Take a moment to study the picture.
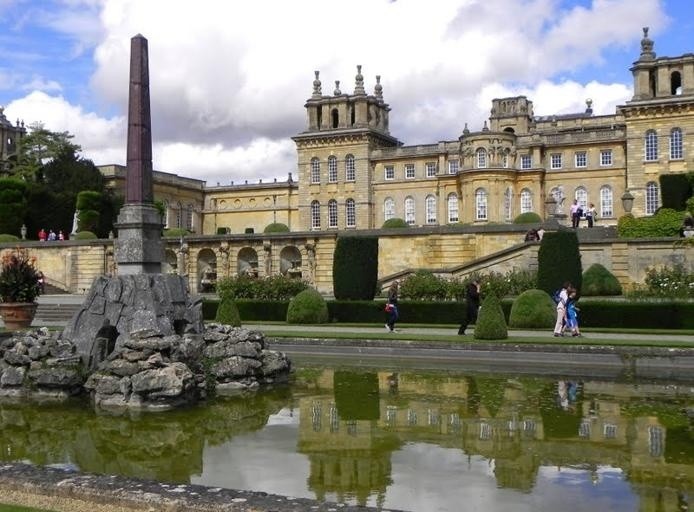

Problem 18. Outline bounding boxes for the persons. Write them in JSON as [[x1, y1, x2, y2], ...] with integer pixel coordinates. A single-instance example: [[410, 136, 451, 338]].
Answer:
[[553, 280, 580, 337], [559, 287, 581, 337], [464, 375, 481, 420], [569, 199, 582, 227], [384, 280, 401, 334], [46, 229, 56, 241], [534, 228, 546, 242], [457, 277, 481, 335], [385, 371, 400, 398], [556, 379, 570, 412], [567, 380, 579, 415], [37, 229, 46, 241], [585, 204, 598, 227], [56, 230, 64, 241], [35, 270, 46, 296], [524, 228, 540, 243]]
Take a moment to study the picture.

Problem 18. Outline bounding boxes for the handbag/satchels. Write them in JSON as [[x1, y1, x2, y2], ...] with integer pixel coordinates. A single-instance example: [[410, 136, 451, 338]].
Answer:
[[385, 303, 394, 313]]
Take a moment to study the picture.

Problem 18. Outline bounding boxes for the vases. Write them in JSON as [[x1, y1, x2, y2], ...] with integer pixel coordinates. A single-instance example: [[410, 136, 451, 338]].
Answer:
[[0, 304, 39, 330]]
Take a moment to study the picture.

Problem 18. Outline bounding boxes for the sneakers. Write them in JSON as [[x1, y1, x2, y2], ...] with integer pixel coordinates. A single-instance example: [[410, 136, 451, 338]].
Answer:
[[554, 332, 581, 337], [385, 323, 398, 333]]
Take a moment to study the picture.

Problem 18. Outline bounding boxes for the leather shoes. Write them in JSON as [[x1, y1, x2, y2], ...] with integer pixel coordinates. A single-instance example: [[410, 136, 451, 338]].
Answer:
[[458, 332, 465, 335]]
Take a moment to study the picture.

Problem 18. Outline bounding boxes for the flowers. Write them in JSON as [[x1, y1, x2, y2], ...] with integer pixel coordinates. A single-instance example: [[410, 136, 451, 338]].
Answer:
[[0, 245, 44, 302]]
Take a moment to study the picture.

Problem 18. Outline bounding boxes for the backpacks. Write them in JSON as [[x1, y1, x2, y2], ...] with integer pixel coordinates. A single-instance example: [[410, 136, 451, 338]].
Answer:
[[552, 288, 562, 304]]
